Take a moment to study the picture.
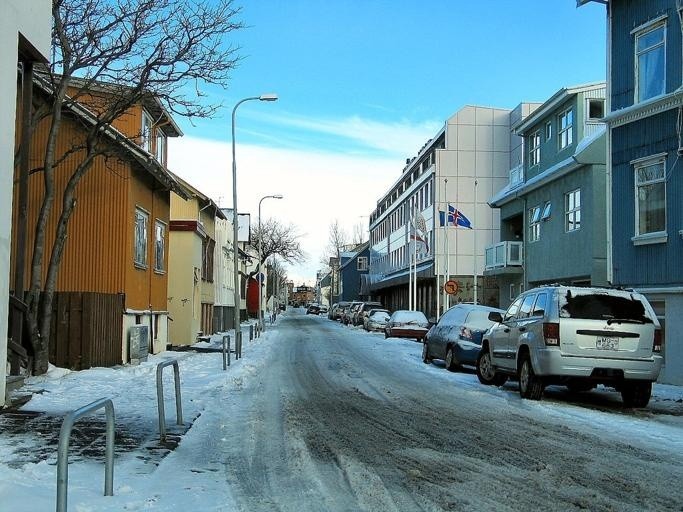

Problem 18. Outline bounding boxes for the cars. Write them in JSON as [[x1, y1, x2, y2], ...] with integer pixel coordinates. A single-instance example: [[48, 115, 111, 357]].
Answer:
[[292, 301, 299, 308], [279, 302, 286, 310], [307, 303, 327, 315]]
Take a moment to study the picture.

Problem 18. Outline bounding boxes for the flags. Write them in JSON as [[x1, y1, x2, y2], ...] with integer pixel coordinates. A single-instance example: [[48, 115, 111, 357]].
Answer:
[[408, 222, 425, 242], [439, 212, 453, 227], [414, 208, 429, 253], [446, 204, 472, 229]]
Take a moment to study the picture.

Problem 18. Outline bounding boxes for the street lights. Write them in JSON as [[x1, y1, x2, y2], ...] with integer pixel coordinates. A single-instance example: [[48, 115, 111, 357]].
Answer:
[[231, 96, 277, 352], [259, 195, 282, 324]]
